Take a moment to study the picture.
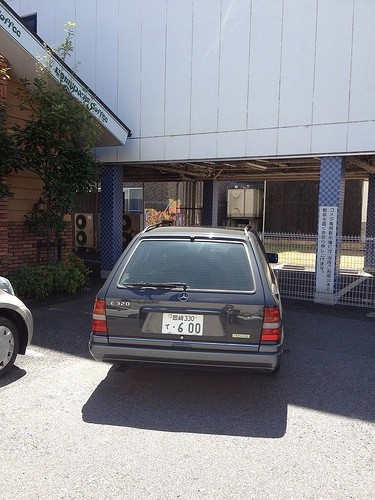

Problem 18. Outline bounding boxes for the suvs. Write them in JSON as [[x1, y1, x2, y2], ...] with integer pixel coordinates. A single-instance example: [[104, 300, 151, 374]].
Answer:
[[88, 222, 284, 375]]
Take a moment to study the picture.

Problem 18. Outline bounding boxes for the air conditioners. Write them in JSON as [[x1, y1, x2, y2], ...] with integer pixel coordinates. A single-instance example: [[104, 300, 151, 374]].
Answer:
[[73, 211, 96, 251]]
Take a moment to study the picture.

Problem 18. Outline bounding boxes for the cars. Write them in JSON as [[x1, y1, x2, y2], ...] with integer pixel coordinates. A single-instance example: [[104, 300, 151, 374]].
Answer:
[[0, 276, 34, 378]]
